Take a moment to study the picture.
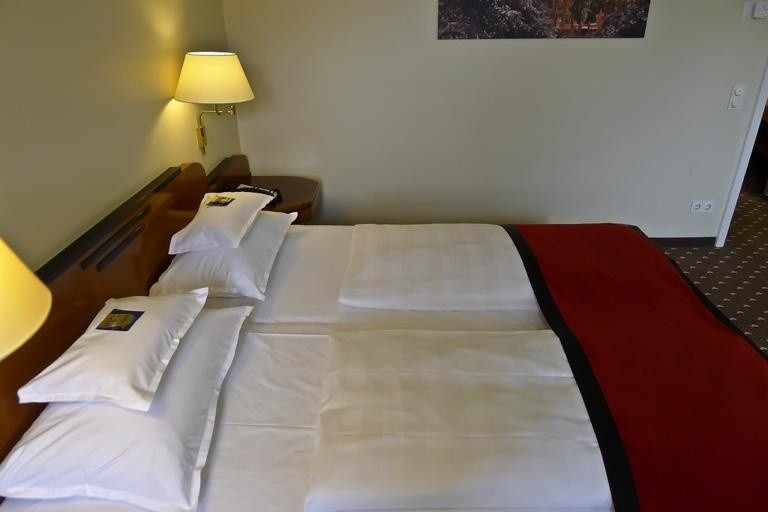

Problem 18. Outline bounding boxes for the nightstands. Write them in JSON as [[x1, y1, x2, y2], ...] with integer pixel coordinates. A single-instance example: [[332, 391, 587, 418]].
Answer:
[[207, 155, 322, 225]]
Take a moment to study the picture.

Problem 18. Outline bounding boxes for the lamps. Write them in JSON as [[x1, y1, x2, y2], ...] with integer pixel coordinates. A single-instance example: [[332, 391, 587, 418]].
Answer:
[[172, 52, 257, 156], [0, 239, 52, 361]]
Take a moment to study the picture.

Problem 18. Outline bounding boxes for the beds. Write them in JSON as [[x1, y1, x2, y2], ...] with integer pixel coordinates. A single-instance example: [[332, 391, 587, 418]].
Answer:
[[0, 162, 768, 511]]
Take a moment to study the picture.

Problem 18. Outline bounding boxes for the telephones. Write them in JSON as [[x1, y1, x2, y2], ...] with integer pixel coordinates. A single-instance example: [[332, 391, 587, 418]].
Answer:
[[223, 182, 283, 207]]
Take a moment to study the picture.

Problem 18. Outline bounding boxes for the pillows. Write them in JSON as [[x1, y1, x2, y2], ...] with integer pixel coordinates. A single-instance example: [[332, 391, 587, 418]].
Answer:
[[170, 192, 275, 254], [0, 306, 256, 512], [149, 213, 294, 299], [15, 283, 209, 415]]
